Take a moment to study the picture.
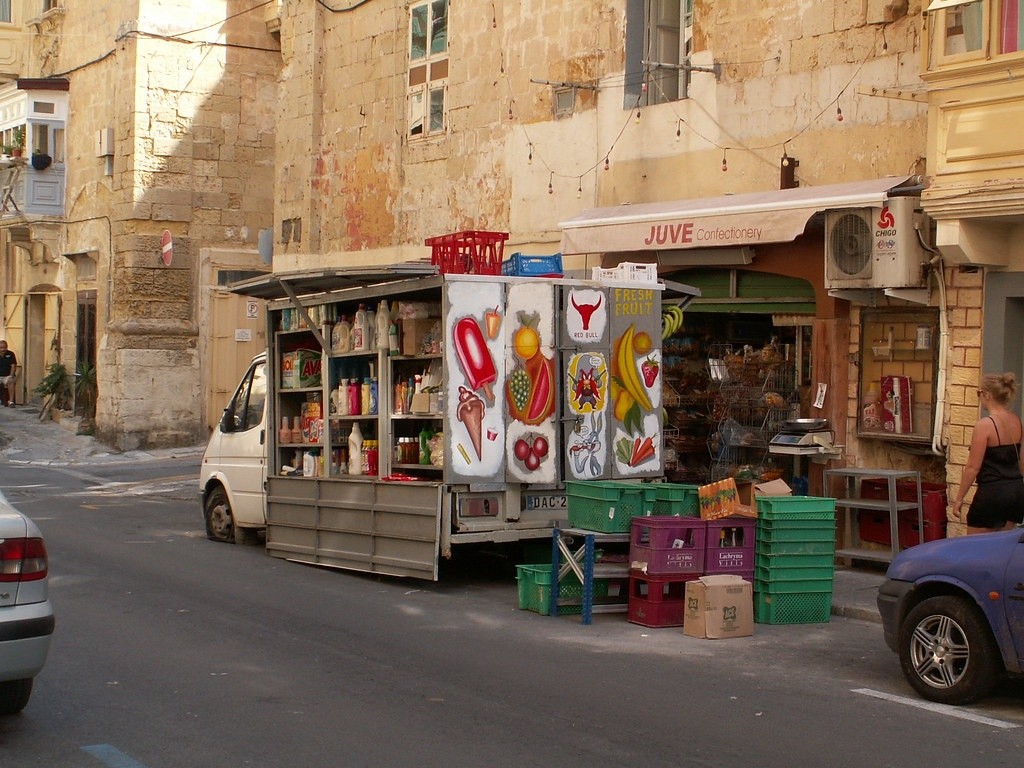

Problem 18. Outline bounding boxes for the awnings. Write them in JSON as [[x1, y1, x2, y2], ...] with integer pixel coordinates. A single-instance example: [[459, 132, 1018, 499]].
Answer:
[[559, 176, 917, 255], [215, 264, 439, 355]]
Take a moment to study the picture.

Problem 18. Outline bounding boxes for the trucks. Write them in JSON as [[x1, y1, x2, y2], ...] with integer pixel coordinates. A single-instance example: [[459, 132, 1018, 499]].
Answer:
[[198, 265, 701, 582]]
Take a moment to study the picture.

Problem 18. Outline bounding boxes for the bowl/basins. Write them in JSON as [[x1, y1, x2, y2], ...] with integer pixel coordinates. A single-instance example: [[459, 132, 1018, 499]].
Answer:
[[780, 418, 827, 430]]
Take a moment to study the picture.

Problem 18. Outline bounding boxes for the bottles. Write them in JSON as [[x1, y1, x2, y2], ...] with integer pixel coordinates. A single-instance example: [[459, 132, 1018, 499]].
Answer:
[[863, 383, 881, 430], [398, 437, 419, 464], [888, 326, 895, 349], [280, 416, 302, 443], [419, 428, 435, 464], [389, 319, 399, 356], [361, 440, 378, 476], [332, 450, 348, 473]]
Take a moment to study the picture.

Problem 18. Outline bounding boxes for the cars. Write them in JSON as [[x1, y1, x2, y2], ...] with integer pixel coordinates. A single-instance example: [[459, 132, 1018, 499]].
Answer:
[[876, 527, 1024, 704], [0, 487, 55, 713]]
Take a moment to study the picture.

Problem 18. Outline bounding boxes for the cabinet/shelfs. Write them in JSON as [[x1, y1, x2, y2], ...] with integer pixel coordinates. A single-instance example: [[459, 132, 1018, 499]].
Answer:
[[663, 335, 813, 483], [274, 312, 443, 479], [823, 467, 924, 563], [551, 528, 650, 625]]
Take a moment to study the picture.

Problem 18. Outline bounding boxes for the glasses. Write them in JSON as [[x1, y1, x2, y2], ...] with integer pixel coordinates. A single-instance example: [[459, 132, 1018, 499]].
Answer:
[[977, 390, 993, 401]]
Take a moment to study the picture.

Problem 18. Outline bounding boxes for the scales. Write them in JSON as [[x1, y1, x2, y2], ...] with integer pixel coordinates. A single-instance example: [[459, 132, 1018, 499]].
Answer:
[[769, 418, 834, 446]]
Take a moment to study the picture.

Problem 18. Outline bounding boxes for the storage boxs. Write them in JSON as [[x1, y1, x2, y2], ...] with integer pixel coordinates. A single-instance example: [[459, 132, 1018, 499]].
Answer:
[[280, 351, 321, 388], [563, 480, 654, 533], [502, 252, 564, 276], [629, 516, 705, 575], [638, 483, 700, 516], [424, 230, 509, 274], [411, 392, 438, 416], [398, 319, 437, 355], [626, 569, 684, 627], [516, 564, 609, 616], [750, 479, 838, 624], [300, 401, 323, 441], [690, 517, 754, 579], [519, 548, 605, 566], [592, 263, 657, 283], [685, 573, 754, 638]]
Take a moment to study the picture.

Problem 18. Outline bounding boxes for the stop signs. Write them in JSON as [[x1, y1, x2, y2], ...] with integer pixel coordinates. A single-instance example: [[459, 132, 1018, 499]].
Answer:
[[161, 230, 173, 266]]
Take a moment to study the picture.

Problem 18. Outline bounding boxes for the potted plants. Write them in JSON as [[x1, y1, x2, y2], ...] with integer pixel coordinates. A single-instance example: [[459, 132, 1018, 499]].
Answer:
[[12, 128, 25, 156], [31, 364, 74, 423], [2, 145, 12, 161], [72, 362, 96, 435]]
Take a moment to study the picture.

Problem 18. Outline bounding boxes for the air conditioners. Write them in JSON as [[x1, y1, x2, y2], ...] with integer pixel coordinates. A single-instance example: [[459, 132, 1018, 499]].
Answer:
[[825, 195, 930, 287]]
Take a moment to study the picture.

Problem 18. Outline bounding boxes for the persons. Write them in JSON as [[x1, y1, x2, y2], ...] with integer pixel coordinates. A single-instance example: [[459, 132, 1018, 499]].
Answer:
[[953, 371, 1024, 536], [0, 339, 17, 408]]
